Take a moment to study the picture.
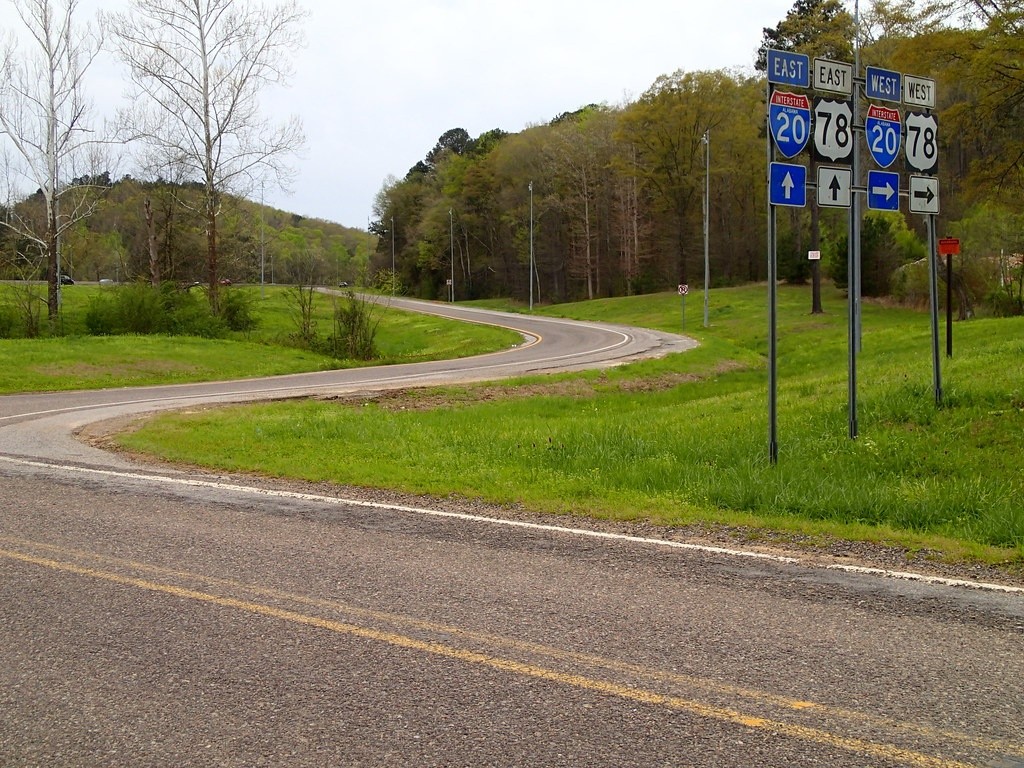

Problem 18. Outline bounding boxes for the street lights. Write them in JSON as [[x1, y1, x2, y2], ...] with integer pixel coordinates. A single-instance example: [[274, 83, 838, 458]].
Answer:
[[390, 216, 396, 297], [526, 180, 536, 311], [700, 129, 711, 328], [447, 206, 455, 303]]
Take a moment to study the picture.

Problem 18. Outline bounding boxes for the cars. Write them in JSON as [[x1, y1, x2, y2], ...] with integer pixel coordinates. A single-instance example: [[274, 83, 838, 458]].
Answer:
[[61, 276, 75, 285], [220, 279, 232, 287], [340, 282, 347, 287], [99, 279, 113, 285]]
